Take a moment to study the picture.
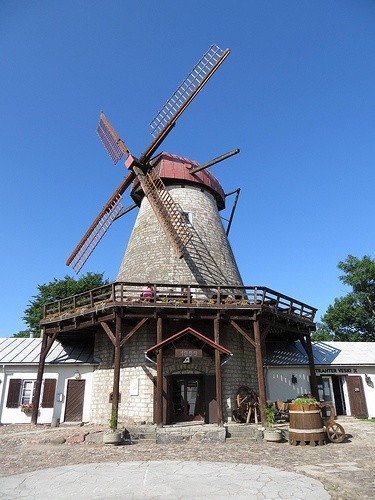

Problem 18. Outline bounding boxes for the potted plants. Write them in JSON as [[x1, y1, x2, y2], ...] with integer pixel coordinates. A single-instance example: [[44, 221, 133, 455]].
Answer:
[[289, 397, 321, 412], [263, 406, 282, 441], [102, 409, 122, 444]]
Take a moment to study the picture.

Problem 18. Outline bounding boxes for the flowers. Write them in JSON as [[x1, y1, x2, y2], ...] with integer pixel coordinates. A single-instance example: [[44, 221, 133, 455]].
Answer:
[[20, 403, 33, 412]]
